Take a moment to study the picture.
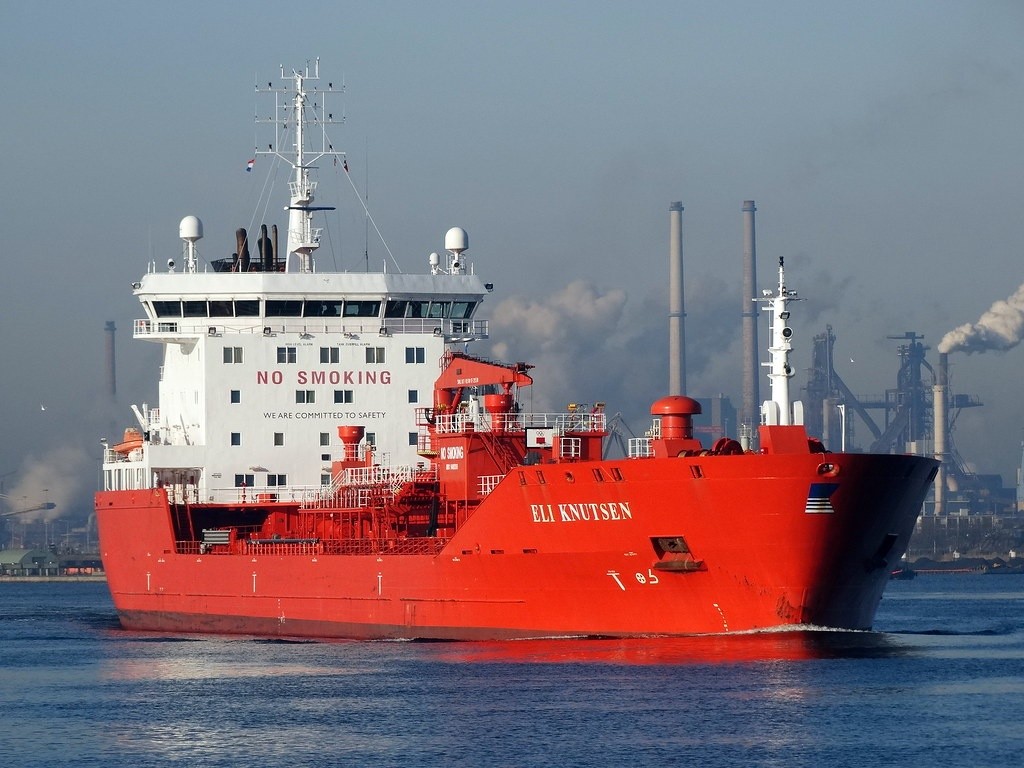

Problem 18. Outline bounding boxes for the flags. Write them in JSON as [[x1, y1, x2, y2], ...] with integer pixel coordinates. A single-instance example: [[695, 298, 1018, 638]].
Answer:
[[246, 159, 254, 172]]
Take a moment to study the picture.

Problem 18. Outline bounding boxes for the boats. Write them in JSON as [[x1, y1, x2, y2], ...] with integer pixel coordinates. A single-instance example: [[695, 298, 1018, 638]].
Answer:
[[91, 56, 942, 644]]
